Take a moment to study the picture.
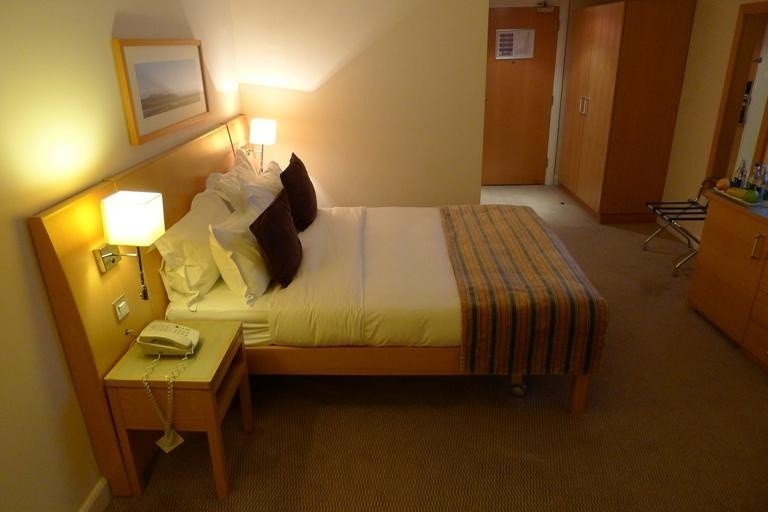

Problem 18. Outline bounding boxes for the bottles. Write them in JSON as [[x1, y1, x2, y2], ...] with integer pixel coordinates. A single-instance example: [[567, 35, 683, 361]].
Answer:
[[732, 159, 767, 201]]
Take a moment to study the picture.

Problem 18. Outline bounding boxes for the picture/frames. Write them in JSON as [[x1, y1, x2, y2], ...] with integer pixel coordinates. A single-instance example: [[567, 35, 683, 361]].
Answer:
[[494, 28, 536, 62], [108, 36, 215, 146]]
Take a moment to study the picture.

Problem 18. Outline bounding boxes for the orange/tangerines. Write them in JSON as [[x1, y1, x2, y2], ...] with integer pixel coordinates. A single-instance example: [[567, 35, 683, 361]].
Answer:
[[744, 190, 759, 202]]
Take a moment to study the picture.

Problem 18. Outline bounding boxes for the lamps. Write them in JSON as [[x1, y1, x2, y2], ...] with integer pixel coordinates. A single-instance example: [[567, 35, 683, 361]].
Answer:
[[91, 189, 170, 301], [248, 116, 280, 175]]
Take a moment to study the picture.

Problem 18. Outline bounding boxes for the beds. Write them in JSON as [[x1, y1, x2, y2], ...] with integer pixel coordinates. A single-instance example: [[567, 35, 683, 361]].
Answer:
[[26, 115, 611, 496]]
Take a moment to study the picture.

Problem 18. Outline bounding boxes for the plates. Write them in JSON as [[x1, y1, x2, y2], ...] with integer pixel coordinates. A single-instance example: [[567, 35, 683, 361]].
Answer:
[[713, 186, 760, 208]]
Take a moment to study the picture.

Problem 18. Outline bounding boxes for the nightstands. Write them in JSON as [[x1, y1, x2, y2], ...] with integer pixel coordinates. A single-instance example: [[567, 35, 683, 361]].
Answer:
[[102, 319, 260, 501]]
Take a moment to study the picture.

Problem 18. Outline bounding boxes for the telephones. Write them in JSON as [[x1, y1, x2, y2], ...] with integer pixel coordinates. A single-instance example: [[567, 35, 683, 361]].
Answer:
[[137, 320, 200, 356]]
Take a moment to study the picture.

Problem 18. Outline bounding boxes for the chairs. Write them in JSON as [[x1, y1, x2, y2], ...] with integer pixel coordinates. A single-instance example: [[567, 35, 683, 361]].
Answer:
[[644, 176, 724, 276]]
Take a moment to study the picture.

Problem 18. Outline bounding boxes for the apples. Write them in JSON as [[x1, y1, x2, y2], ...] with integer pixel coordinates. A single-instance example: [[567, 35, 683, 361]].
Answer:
[[716, 178, 730, 190]]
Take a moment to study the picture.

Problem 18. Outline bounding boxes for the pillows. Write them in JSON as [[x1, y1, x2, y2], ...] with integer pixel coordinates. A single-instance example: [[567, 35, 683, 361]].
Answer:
[[248, 187, 302, 288], [279, 151, 318, 233], [157, 189, 238, 307], [209, 150, 259, 214], [236, 160, 285, 210], [208, 192, 275, 307]]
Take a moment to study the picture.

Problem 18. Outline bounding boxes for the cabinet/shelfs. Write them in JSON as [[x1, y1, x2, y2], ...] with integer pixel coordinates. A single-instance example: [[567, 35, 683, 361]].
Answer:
[[553, 2, 695, 225], [690, 195, 767, 375]]
